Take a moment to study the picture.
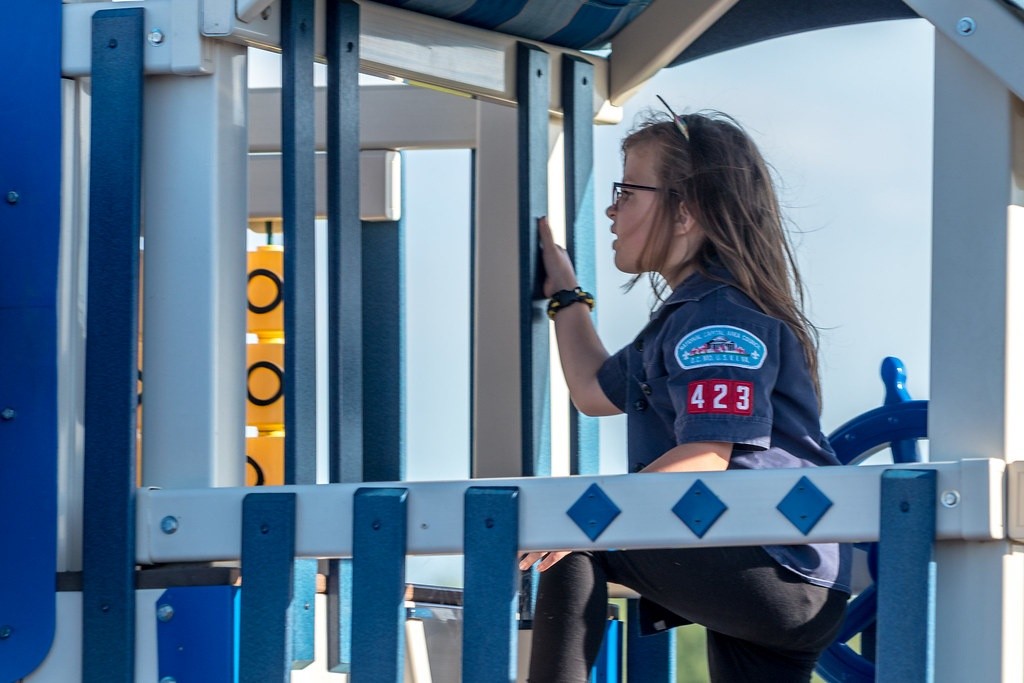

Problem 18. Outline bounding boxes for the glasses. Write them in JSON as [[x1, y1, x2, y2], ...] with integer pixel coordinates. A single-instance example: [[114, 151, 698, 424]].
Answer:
[[612, 182, 683, 206]]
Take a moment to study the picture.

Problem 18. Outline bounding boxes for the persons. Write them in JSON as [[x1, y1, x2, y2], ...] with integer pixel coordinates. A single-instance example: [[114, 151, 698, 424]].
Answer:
[[519, 110, 853, 683]]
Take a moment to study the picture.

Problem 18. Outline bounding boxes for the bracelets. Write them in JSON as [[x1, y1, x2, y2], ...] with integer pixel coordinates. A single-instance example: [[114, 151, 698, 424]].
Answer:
[[546, 286, 594, 320]]
